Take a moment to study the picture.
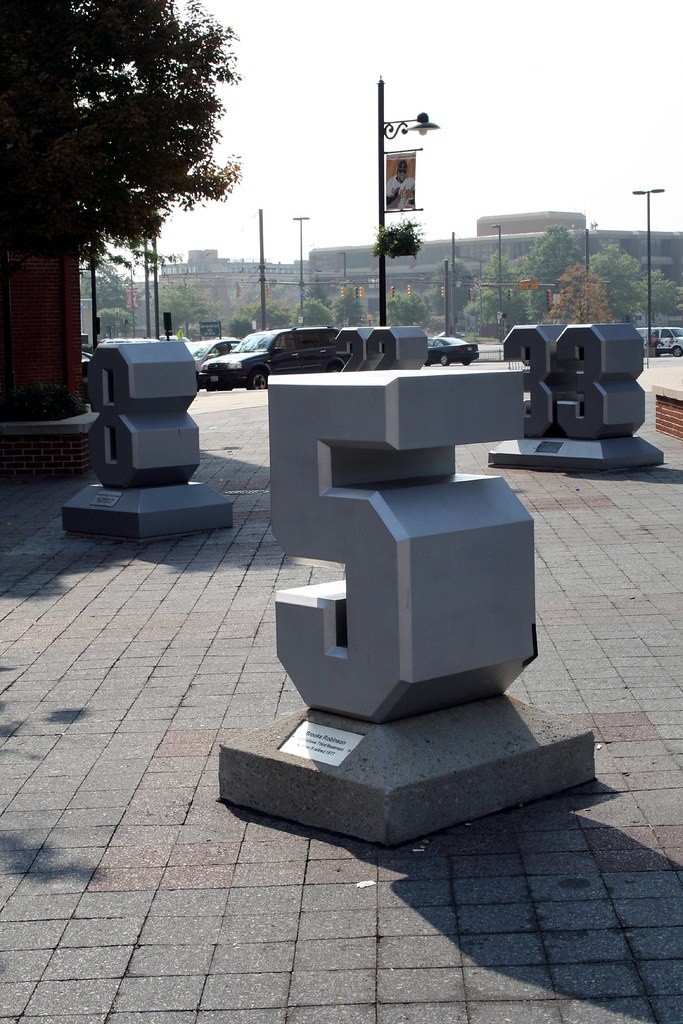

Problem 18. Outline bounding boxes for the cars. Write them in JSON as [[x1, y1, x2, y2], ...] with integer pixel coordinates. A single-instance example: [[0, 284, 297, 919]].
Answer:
[[424, 336, 480, 368], [188, 338, 243, 393]]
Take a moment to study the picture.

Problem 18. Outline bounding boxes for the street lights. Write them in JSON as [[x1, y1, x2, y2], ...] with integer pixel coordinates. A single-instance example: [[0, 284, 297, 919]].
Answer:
[[632, 188, 665, 357], [293, 216, 310, 326], [376, 72, 440, 354], [492, 223, 501, 344]]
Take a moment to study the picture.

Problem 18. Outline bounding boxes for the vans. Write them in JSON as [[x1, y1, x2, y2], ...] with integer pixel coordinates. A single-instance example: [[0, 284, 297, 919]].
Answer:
[[636, 326, 683, 358]]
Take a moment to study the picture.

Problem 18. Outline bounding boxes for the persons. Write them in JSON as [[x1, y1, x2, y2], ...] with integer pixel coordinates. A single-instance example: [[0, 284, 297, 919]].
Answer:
[[386, 160, 415, 209]]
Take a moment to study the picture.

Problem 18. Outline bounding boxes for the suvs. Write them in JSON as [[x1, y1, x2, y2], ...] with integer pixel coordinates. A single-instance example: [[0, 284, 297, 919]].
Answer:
[[199, 324, 353, 391]]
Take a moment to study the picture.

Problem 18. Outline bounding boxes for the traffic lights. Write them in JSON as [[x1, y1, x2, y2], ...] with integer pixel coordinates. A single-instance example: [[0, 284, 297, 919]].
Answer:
[[353, 286, 359, 299], [390, 285, 396, 298], [546, 288, 553, 302], [469, 288, 474, 300], [440, 286, 445, 297], [341, 287, 347, 299], [508, 288, 513, 301], [407, 283, 413, 295]]
